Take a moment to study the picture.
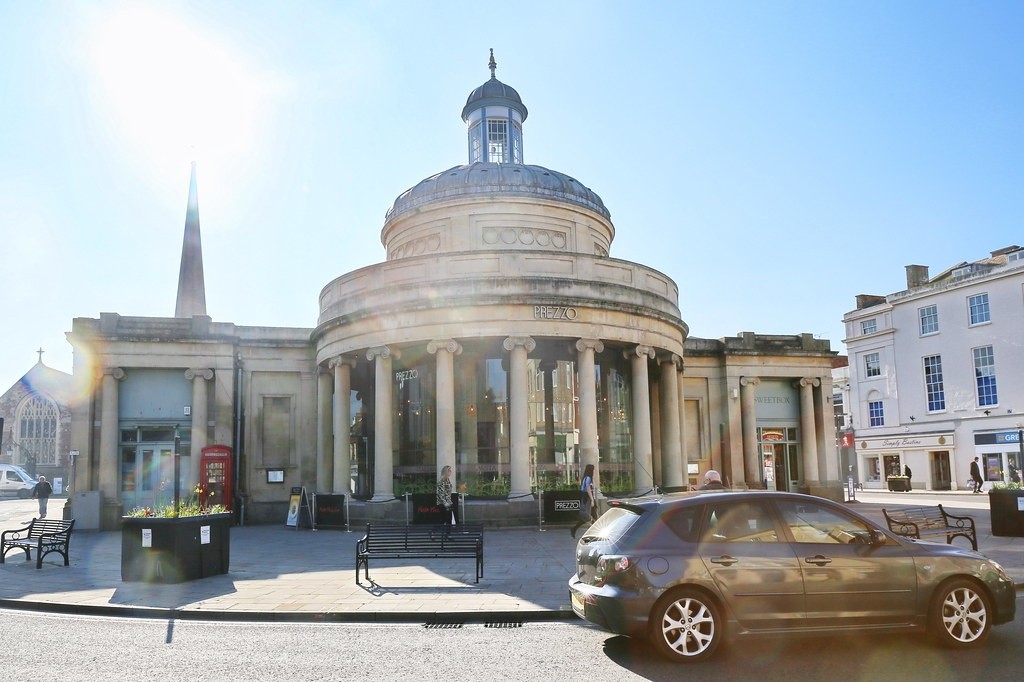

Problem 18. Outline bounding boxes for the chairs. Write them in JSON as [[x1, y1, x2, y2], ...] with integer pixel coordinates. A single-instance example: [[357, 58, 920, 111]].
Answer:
[[0, 518, 75, 569], [882, 504, 978, 551], [356, 523, 483, 585]]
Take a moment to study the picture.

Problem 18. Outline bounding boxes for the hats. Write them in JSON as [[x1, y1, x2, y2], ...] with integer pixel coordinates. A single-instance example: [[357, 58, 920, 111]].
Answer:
[[974, 457, 979, 460], [1008, 458, 1013, 463]]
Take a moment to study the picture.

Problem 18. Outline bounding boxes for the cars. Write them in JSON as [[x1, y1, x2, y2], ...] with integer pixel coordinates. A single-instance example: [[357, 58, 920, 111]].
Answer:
[[568, 489, 1016, 664]]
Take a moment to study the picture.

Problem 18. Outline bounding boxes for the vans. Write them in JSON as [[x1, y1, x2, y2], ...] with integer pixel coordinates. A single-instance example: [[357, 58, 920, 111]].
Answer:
[[0, 464, 38, 499]]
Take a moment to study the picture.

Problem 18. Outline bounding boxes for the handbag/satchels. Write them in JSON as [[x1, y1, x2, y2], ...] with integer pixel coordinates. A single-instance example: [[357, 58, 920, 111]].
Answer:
[[580, 497, 591, 521], [967, 479, 975, 488], [451, 511, 456, 528]]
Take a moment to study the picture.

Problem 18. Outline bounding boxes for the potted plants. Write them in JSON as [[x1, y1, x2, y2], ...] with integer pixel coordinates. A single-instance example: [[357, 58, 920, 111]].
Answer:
[[887, 475, 910, 492]]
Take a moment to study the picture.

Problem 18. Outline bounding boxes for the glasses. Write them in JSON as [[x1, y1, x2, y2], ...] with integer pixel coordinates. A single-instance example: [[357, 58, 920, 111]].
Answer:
[[704, 479, 710, 484]]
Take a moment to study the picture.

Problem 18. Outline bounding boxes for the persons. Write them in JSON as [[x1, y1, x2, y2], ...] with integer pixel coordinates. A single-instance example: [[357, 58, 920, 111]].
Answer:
[[427, 466, 456, 541], [764, 455, 772, 467], [970, 457, 983, 493], [570, 464, 597, 539], [32, 476, 53, 520], [696, 470, 736, 529], [905, 465, 912, 490], [1008, 458, 1020, 489]]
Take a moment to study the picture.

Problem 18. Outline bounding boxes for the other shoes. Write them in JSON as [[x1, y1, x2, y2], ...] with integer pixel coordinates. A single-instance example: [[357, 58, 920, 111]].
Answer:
[[973, 491, 979, 493], [977, 490, 983, 492], [570, 526, 575, 539], [428, 530, 435, 540], [445, 536, 454, 541], [41, 515, 46, 518]]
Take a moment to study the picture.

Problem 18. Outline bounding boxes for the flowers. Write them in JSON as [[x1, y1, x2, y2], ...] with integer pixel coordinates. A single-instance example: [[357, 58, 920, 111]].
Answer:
[[126, 480, 229, 518], [988, 467, 1024, 491]]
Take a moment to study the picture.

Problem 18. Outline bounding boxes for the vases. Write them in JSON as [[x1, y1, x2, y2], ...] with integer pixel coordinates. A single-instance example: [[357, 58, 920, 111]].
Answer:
[[120, 511, 233, 585], [988, 489, 1024, 537]]
